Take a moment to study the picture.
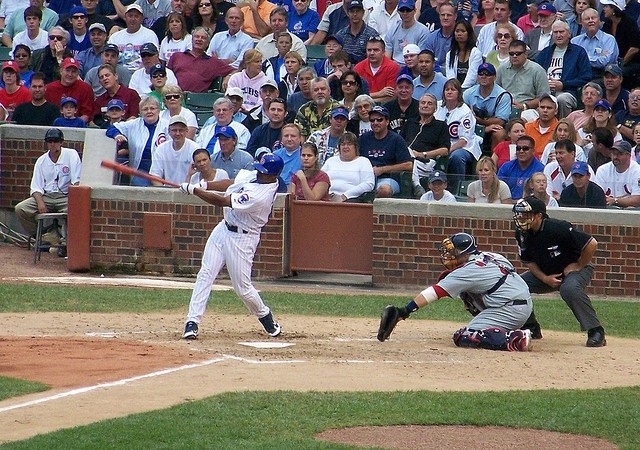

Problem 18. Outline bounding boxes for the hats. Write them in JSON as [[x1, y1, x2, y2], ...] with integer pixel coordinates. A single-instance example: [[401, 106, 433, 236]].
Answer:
[[103, 44, 118, 53], [2, 62, 19, 73], [150, 64, 165, 74], [124, 4, 143, 14], [368, 106, 389, 116], [403, 44, 421, 56], [537, 4, 556, 15], [539, 92, 558, 109], [61, 97, 77, 106], [605, 64, 622, 75], [600, 0, 626, 11], [397, 0, 415, 10], [593, 100, 609, 110], [214, 127, 235, 137], [225, 88, 243, 98], [331, 108, 349, 118], [108, 100, 124, 110], [428, 170, 446, 182], [324, 33, 343, 47], [349, 0, 364, 10], [141, 43, 158, 54], [89, 23, 106, 32], [260, 79, 278, 90], [70, 6, 87, 15], [63, 58, 79, 68], [478, 63, 496, 76], [169, 116, 187, 127], [571, 162, 587, 175], [397, 74, 413, 84], [609, 142, 631, 153]]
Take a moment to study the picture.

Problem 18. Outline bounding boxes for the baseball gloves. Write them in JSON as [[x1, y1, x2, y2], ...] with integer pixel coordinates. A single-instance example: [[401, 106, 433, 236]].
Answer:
[[377, 304, 398, 342]]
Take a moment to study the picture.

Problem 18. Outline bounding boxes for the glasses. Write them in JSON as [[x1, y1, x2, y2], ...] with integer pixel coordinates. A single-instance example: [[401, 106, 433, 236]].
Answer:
[[509, 52, 524, 55], [343, 81, 356, 86], [152, 73, 164, 78], [198, 2, 211, 7], [497, 33, 510, 39], [15, 54, 27, 58], [72, 15, 85, 19], [370, 118, 383, 122], [629, 94, 640, 99], [165, 95, 180, 100], [516, 146, 529, 151], [369, 35, 381, 40], [355, 104, 369, 112], [48, 35, 63, 41]]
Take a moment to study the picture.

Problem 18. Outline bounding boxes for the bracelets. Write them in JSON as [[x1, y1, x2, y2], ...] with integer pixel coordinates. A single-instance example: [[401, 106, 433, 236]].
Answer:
[[616, 123, 622, 129], [523, 103, 529, 110], [615, 198, 618, 206], [407, 299, 419, 314], [228, 73, 232, 76], [227, 73, 233, 76]]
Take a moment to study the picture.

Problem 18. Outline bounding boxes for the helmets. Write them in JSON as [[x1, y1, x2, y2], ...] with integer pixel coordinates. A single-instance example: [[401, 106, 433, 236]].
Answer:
[[438, 233, 478, 269], [512, 196, 546, 237], [44, 128, 63, 140], [252, 155, 284, 175]]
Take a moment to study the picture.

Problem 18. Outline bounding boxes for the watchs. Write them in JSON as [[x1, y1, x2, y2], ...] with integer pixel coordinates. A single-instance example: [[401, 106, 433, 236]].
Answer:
[[423, 151, 427, 159], [56, 60, 62, 63]]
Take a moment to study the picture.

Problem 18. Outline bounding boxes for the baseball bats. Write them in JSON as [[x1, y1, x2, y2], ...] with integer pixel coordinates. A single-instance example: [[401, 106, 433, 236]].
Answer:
[[100, 159, 181, 189]]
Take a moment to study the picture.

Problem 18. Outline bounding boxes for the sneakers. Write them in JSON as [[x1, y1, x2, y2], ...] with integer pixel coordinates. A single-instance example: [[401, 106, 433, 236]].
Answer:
[[515, 329, 531, 351], [183, 321, 198, 339], [587, 326, 606, 347], [258, 311, 282, 337], [521, 323, 542, 339]]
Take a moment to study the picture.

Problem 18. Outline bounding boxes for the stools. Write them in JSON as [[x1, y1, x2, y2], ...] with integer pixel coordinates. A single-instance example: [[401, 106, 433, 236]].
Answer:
[[32, 211, 68, 265]]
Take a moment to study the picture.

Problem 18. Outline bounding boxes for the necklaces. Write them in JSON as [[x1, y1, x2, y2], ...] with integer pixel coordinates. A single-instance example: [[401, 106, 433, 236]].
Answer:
[[344, 99, 354, 109]]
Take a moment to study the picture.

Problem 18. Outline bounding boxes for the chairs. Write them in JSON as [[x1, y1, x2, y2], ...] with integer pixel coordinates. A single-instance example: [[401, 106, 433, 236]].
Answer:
[[0, 47, 12, 74], [183, 89, 224, 134], [305, 42, 327, 67], [209, 76, 223, 92], [454, 180, 473, 202]]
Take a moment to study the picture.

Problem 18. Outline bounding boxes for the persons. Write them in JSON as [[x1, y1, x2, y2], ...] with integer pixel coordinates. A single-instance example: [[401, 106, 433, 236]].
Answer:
[[433, 78, 483, 187], [312, 34, 344, 78], [0, 0, 60, 48], [570, 8, 620, 89], [552, 0, 574, 22], [384, 0, 430, 69], [308, 0, 343, 21], [630, 121, 640, 163], [88, 63, 141, 127], [277, 50, 302, 101], [378, 233, 533, 352], [536, 20, 593, 121], [0, 60, 32, 120], [497, 135, 545, 204], [396, 44, 443, 81], [484, 23, 517, 69], [55, 0, 116, 36], [198, 87, 248, 130], [106, 4, 160, 73], [64, 6, 93, 58], [419, 170, 457, 203], [112, 0, 173, 29], [0, 43, 34, 89], [578, 99, 624, 161], [127, 43, 178, 100], [476, 0, 525, 58], [97, 0, 136, 28], [368, 0, 402, 39], [273, 122, 303, 184], [149, 0, 193, 45], [595, 141, 640, 207], [490, 118, 527, 175], [159, 11, 193, 67], [136, 63, 186, 117], [615, 88, 640, 147], [262, 32, 293, 83], [362, 0, 385, 14], [352, 36, 402, 102], [212, 1, 236, 23], [517, 0, 549, 36], [466, 156, 511, 204], [0, 0, 50, 29], [53, 97, 87, 128], [240, 78, 297, 134], [225, 49, 269, 111], [602, 63, 631, 114], [188, 0, 228, 51], [600, 0, 640, 90], [254, 7, 307, 66], [381, 74, 420, 135], [11, 72, 60, 126], [148, 114, 202, 187], [540, 118, 587, 163], [160, 84, 199, 141], [246, 98, 289, 159], [565, 82, 603, 133], [28, 26, 74, 83], [10, 6, 48, 61], [507, 0, 531, 24], [587, 127, 614, 175], [334, 0, 381, 64], [398, 92, 451, 196], [455, 0, 479, 30], [522, 172, 559, 207], [207, 126, 255, 180], [336, 70, 362, 120], [557, 161, 606, 209], [43, 56, 94, 124], [75, 23, 123, 81], [100, 99, 127, 129], [84, 44, 132, 101], [524, 4, 557, 63], [194, 97, 251, 156], [179, 153, 285, 338], [287, 0, 320, 46], [346, 94, 377, 139], [476, 0, 498, 24], [289, 142, 331, 201], [517, 196, 606, 347], [415, 0, 438, 22], [462, 62, 513, 156], [524, 93, 559, 161], [310, 1, 370, 44], [634, 143, 640, 165], [237, 0, 280, 40], [294, 76, 342, 146], [286, 66, 333, 114], [167, 26, 239, 93], [320, 132, 376, 202], [412, 49, 449, 101], [45, 0, 83, 21], [566, 1, 603, 39], [417, 0, 465, 33], [184, 148, 230, 192], [494, 39, 551, 124], [105, 96, 173, 187], [15, 128, 81, 256], [205, 7, 254, 70], [304, 107, 349, 170], [252, 147, 287, 193], [543, 139, 596, 200], [597, 0, 640, 27], [325, 50, 369, 101], [446, 19, 484, 89], [424, 2, 458, 77], [357, 105, 414, 198]]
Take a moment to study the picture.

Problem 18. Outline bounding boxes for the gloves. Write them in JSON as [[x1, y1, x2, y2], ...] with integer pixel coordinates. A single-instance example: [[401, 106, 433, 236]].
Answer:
[[194, 179, 207, 190], [180, 183, 195, 195]]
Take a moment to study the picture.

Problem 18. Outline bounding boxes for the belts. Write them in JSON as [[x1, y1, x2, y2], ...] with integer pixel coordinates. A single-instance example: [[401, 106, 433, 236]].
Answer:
[[513, 300, 527, 304], [225, 220, 248, 234]]
